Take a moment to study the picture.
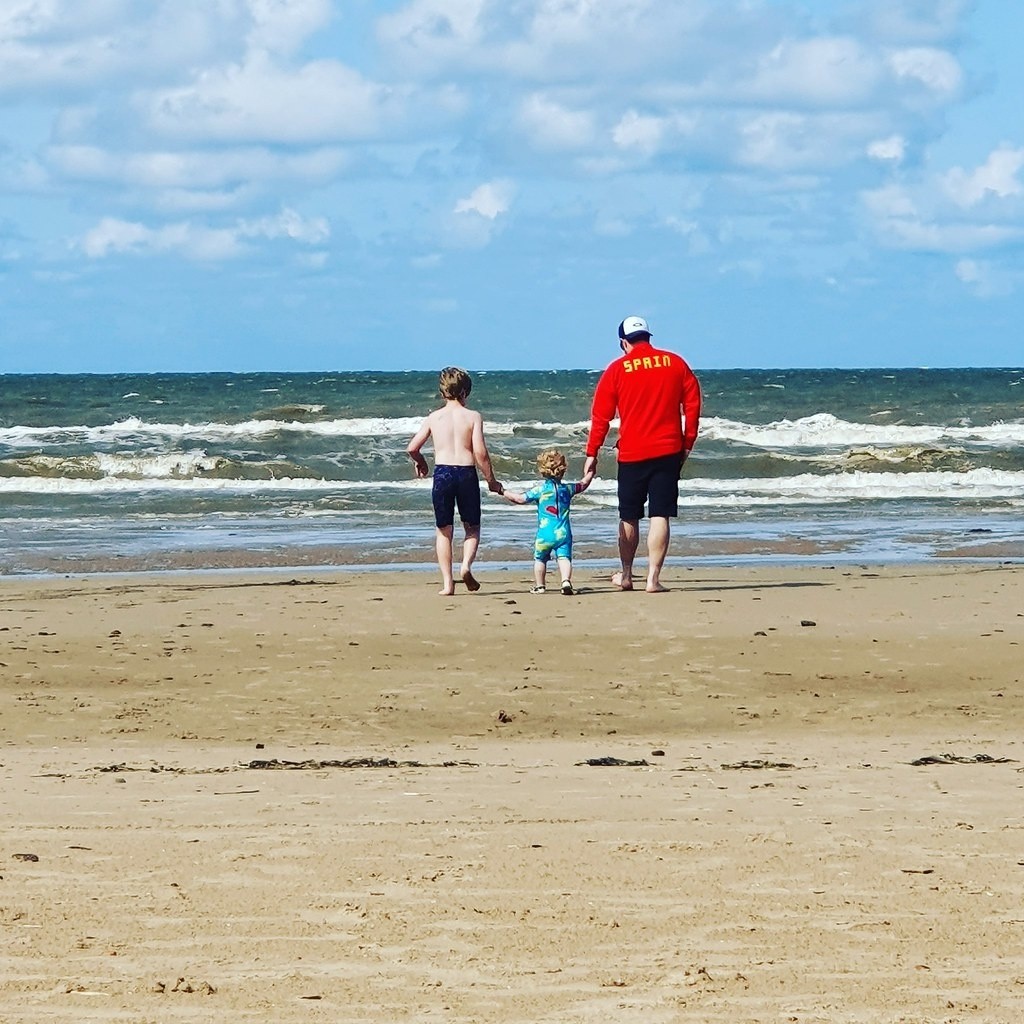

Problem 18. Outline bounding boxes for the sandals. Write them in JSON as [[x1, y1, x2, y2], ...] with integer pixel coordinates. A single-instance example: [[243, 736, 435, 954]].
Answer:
[[530, 586, 545, 594], [560, 580, 574, 595]]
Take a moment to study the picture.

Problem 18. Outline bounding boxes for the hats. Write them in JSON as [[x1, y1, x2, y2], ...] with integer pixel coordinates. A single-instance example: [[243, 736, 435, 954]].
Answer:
[[619, 316, 653, 339]]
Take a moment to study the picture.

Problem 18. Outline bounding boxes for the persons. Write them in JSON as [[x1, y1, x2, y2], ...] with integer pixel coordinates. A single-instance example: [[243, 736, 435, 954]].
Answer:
[[406, 316, 701, 595]]
